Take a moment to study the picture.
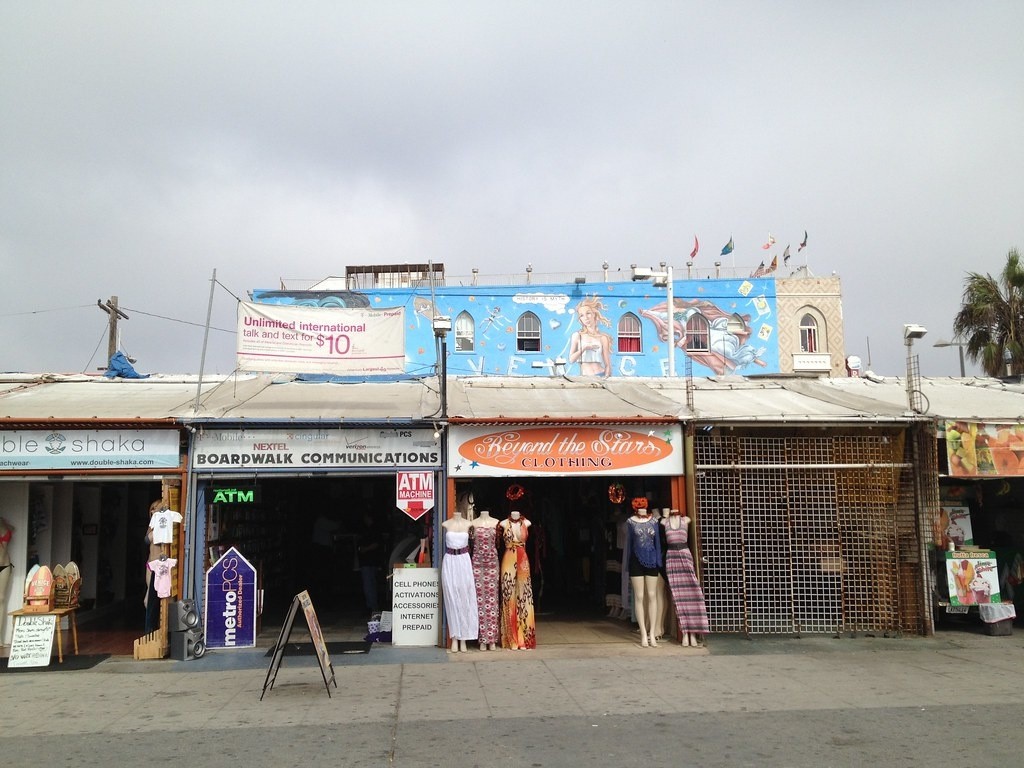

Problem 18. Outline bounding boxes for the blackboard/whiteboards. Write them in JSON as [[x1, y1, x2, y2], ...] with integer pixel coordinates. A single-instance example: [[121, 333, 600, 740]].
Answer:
[[6, 615, 58, 670]]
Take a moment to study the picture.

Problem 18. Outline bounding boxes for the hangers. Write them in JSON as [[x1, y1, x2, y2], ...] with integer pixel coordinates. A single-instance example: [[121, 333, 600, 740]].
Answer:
[[159, 552, 167, 561], [163, 506, 167, 512]]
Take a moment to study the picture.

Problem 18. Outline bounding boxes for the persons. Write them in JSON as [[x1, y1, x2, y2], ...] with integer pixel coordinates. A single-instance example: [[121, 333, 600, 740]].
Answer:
[[618, 514, 630, 620], [306, 507, 392, 611], [500, 510, 537, 650], [604, 513, 622, 618], [648, 507, 679, 638], [635, 508, 668, 638], [441, 512, 479, 652], [0, 516, 15, 649], [661, 510, 699, 647], [144, 499, 163, 609], [471, 510, 499, 651], [627, 509, 660, 648]]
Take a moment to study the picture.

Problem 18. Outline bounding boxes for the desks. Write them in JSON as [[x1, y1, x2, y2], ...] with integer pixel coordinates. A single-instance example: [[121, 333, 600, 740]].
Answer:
[[6, 606, 79, 663]]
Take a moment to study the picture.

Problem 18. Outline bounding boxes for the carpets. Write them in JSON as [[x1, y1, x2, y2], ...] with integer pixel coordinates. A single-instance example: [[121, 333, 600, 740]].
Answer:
[[0, 653, 112, 673], [264, 641, 373, 656]]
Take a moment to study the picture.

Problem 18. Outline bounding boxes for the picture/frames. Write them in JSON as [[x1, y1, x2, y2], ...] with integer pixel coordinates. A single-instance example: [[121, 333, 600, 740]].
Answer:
[[83, 523, 97, 535]]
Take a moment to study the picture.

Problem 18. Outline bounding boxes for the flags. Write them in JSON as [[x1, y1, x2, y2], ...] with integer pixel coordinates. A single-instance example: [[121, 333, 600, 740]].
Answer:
[[762, 232, 776, 249], [720, 232, 735, 256], [690, 234, 700, 258], [764, 254, 778, 276], [752, 261, 765, 279], [797, 230, 807, 253], [782, 244, 791, 267]]
